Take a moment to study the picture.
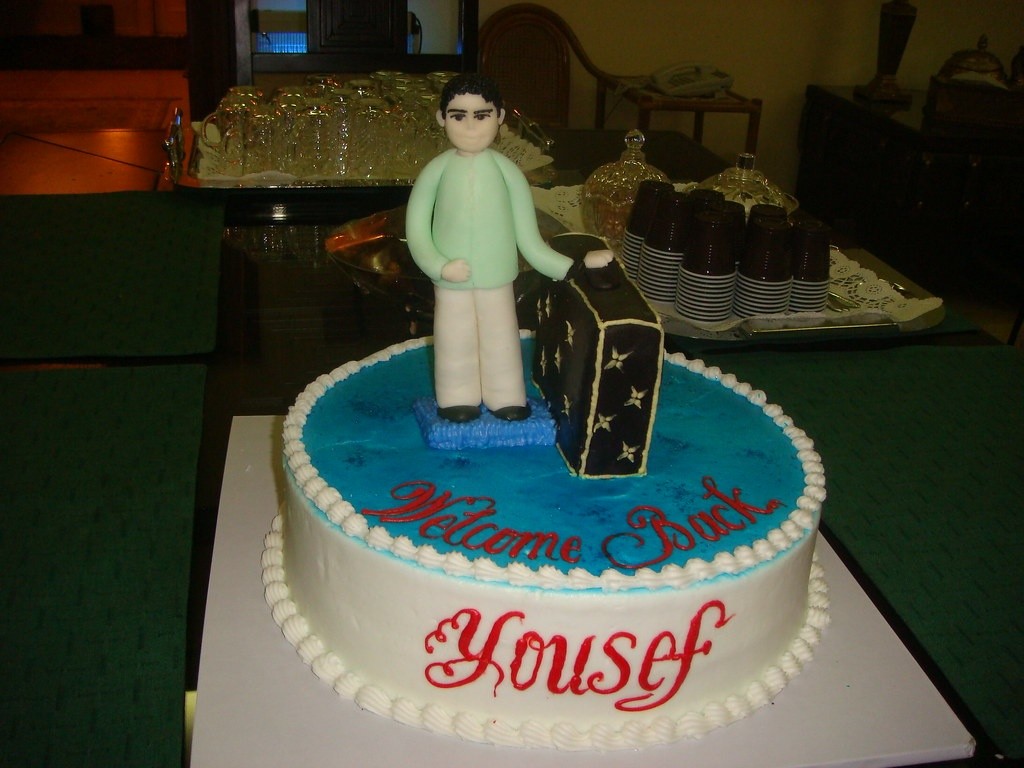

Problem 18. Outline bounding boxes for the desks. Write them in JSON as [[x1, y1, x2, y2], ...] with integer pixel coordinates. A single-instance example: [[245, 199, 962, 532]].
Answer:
[[0, 363, 207, 768], [162, 71, 555, 227], [0, 198, 223, 359], [686, 346, 1024, 757]]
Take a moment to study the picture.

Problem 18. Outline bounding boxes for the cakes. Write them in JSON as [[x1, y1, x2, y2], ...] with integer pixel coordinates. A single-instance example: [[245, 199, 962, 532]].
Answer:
[[262, 231, 830, 750]]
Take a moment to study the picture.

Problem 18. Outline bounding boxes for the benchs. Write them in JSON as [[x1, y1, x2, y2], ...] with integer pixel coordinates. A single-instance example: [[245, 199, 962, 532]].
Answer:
[[0, 68, 190, 195]]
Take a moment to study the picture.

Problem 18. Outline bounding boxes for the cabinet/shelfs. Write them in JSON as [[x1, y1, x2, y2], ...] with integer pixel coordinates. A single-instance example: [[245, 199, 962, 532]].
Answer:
[[793, 85, 1024, 307], [611, 74, 763, 167]]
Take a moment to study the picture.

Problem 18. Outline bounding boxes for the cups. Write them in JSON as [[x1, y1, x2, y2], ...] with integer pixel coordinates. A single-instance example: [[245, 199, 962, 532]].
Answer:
[[619, 181, 830, 321], [203, 69, 471, 176]]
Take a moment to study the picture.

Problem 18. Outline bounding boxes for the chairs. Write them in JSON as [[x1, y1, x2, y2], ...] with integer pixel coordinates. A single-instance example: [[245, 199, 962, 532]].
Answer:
[[479, 3, 646, 128]]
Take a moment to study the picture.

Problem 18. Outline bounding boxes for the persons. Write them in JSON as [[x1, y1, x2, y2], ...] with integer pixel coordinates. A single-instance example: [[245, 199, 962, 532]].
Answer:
[[406, 76, 619, 422]]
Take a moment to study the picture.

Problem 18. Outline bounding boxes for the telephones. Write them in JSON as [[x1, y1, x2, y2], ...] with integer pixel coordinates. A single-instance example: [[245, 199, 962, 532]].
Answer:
[[649, 58, 735, 97]]
